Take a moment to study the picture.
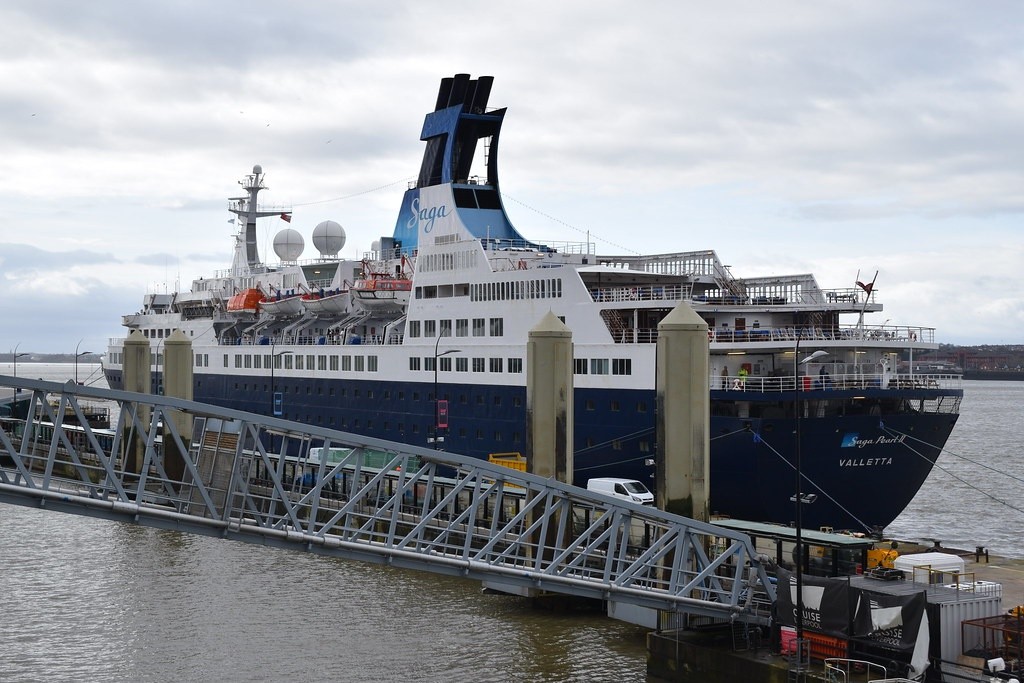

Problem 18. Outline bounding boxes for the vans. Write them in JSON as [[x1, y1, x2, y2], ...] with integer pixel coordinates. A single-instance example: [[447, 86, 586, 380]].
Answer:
[[587, 476, 655, 506]]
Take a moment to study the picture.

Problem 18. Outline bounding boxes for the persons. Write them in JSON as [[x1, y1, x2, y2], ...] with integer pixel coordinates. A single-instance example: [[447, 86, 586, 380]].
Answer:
[[819, 366, 832, 383], [721, 366, 729, 388]]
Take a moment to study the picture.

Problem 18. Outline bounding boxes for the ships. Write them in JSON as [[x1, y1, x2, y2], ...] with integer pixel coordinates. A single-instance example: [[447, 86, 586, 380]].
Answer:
[[95, 72, 967, 527]]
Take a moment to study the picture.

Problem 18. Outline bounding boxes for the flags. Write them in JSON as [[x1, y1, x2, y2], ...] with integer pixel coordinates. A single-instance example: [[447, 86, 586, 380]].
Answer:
[[855, 282, 873, 295], [280, 214, 291, 223]]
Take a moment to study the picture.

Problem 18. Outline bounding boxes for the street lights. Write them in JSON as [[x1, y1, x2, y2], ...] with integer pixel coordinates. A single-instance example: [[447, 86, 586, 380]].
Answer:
[[794, 319, 830, 663], [269, 329, 295, 453], [435, 325, 462, 476], [13, 341, 30, 437], [74, 338, 93, 450]]
